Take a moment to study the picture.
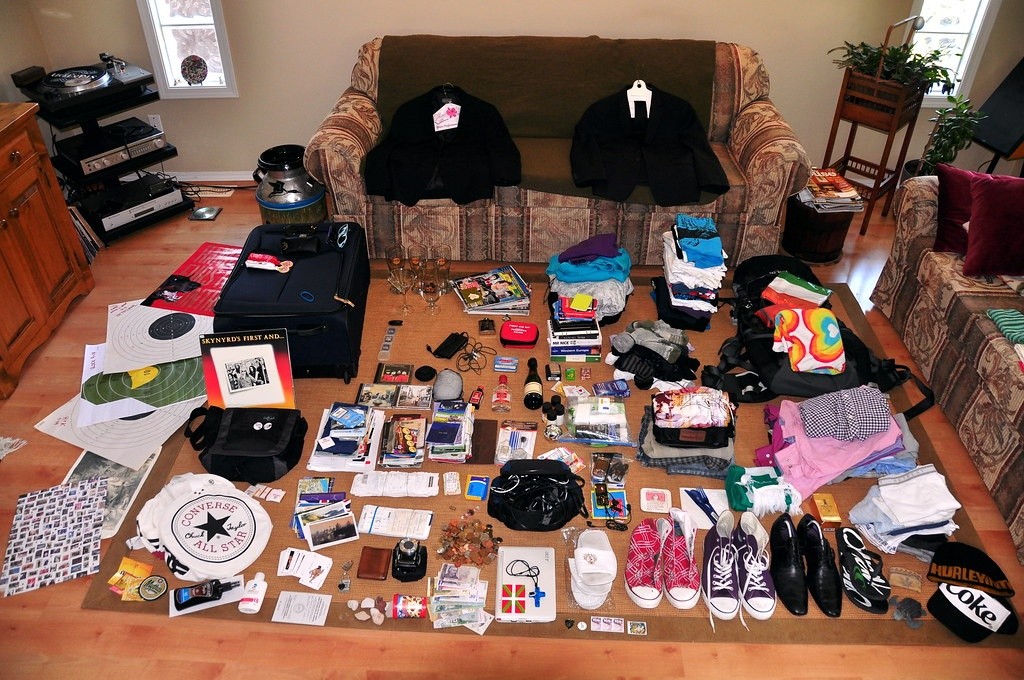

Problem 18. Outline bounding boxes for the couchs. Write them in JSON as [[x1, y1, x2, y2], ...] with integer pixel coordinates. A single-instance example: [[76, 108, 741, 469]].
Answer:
[[869, 174, 1024, 563], [303, 36, 811, 265]]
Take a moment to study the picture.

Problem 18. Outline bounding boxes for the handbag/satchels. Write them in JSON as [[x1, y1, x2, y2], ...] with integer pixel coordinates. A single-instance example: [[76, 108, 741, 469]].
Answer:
[[124, 473, 276, 583], [488, 459, 589, 532], [703, 253, 910, 401], [183, 406, 308, 486]]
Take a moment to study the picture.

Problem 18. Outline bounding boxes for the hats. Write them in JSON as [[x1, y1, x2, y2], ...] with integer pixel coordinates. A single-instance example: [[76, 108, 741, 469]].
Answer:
[[926, 576, 1019, 643], [927, 542, 1015, 597]]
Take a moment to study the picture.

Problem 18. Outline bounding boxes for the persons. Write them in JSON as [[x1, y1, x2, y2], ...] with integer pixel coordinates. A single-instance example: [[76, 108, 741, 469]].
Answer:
[[473, 273, 501, 287], [382, 369, 408, 382], [483, 277, 511, 295], [229, 357, 266, 390], [486, 289, 514, 306], [361, 390, 391, 403]]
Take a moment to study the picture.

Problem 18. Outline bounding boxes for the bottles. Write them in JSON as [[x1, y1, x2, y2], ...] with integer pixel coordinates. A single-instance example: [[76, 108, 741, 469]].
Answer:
[[252, 144, 328, 225], [491, 374, 513, 413], [523, 357, 544, 409]]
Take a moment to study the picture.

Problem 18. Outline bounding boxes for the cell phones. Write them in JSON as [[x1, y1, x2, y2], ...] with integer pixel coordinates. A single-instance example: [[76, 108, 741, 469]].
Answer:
[[594, 483, 608, 505], [591, 458, 610, 481]]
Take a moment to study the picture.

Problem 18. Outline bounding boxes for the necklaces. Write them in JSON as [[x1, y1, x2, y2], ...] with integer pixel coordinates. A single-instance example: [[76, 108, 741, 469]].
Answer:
[[506, 560, 545, 608]]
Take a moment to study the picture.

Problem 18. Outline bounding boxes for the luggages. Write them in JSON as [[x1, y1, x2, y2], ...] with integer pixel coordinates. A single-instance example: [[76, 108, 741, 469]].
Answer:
[[212, 218, 367, 385]]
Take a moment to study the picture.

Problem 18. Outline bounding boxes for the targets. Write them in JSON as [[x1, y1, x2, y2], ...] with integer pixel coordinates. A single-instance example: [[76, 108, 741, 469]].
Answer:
[[80, 341, 208, 426], [34, 392, 207, 471], [103, 299, 214, 375]]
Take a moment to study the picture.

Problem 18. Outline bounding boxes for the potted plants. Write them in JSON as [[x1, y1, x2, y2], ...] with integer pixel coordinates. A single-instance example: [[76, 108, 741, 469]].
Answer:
[[899, 94, 988, 184]]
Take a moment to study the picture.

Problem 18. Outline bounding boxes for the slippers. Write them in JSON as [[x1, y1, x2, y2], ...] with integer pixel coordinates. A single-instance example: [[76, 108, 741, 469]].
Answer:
[[575, 532, 617, 586], [568, 557, 612, 610], [836, 527, 890, 614]]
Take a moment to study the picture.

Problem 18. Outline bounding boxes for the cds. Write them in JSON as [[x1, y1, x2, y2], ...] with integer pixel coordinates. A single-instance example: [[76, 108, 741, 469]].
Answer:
[[194, 207, 215, 219]]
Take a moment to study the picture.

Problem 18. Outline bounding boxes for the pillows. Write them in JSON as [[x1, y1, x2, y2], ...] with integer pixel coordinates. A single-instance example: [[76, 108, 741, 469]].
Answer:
[[936, 162, 1024, 275]]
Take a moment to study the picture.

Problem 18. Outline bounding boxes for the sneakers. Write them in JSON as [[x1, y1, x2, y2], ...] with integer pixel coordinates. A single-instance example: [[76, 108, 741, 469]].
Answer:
[[663, 508, 702, 610], [732, 510, 777, 632], [700, 510, 741, 633], [623, 517, 673, 610]]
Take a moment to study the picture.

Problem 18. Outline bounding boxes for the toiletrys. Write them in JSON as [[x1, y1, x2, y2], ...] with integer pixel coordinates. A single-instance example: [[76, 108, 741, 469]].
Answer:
[[238, 572, 267, 614], [173, 579, 240, 612]]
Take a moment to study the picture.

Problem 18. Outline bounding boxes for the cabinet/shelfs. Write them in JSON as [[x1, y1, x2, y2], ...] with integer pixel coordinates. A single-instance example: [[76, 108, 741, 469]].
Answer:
[[10, 52, 195, 248], [822, 16, 938, 236], [0, 102, 95, 401]]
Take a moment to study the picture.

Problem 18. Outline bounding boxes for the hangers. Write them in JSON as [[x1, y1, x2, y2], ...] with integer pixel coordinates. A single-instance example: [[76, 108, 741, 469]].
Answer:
[[397, 82, 500, 126], [583, 65, 698, 123]]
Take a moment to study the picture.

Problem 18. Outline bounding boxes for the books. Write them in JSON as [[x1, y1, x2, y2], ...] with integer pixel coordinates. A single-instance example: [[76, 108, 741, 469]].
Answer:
[[452, 265, 530, 317], [796, 169, 867, 212]]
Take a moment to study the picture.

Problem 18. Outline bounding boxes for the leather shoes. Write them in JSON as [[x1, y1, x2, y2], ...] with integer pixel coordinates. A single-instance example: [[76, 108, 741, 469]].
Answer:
[[797, 513, 842, 617], [770, 512, 808, 616]]
